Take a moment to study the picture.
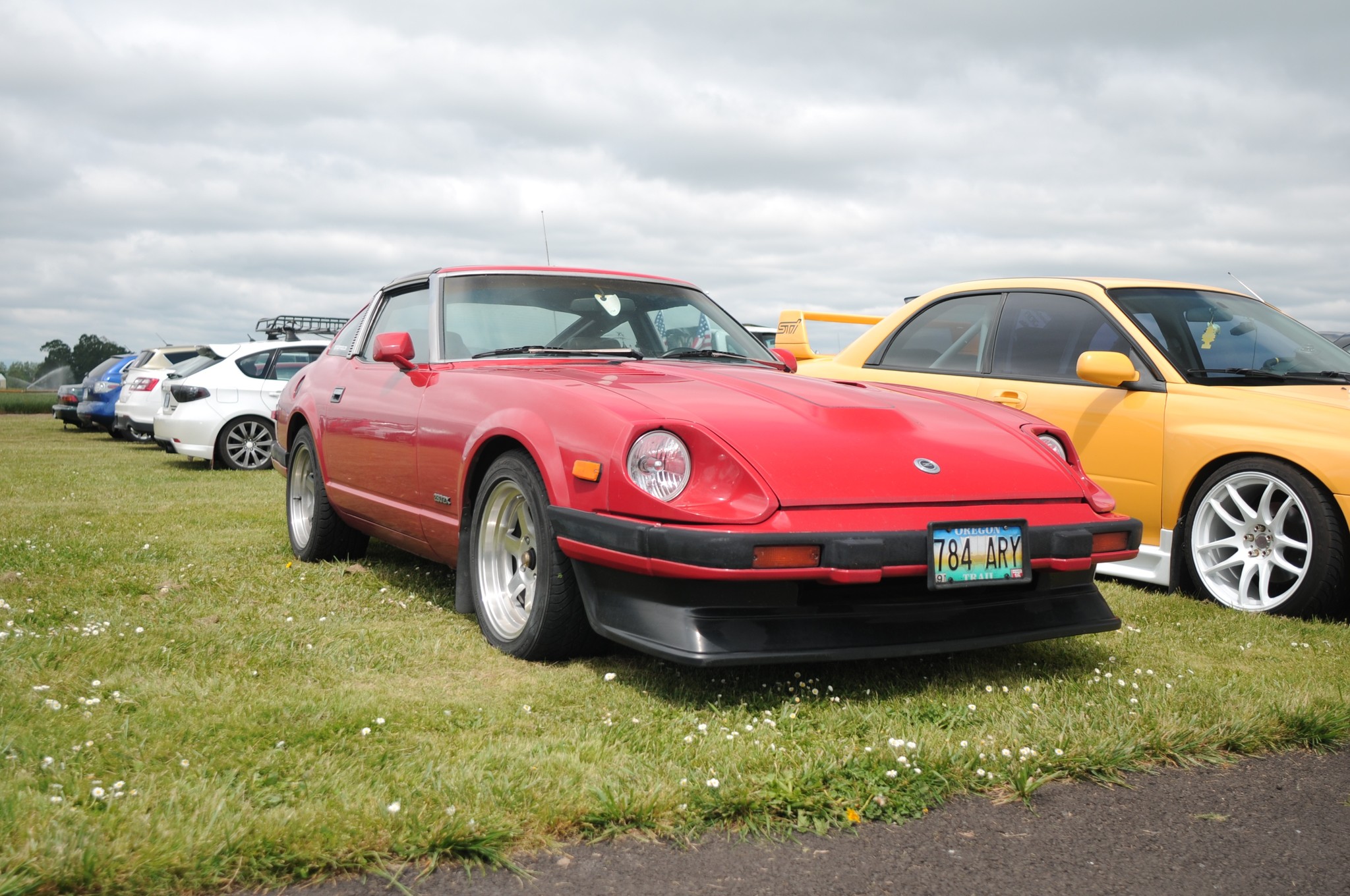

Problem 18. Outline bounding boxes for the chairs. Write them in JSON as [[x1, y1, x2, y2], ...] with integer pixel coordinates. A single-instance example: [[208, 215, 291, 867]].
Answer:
[[568, 337, 620, 349], [406, 329, 472, 364], [242, 362, 259, 376]]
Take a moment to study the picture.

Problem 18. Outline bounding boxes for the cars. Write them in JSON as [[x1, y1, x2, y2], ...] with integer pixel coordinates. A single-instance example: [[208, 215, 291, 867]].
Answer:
[[50, 322, 778, 474], [775, 273, 1350, 623], [267, 265, 1144, 672]]
